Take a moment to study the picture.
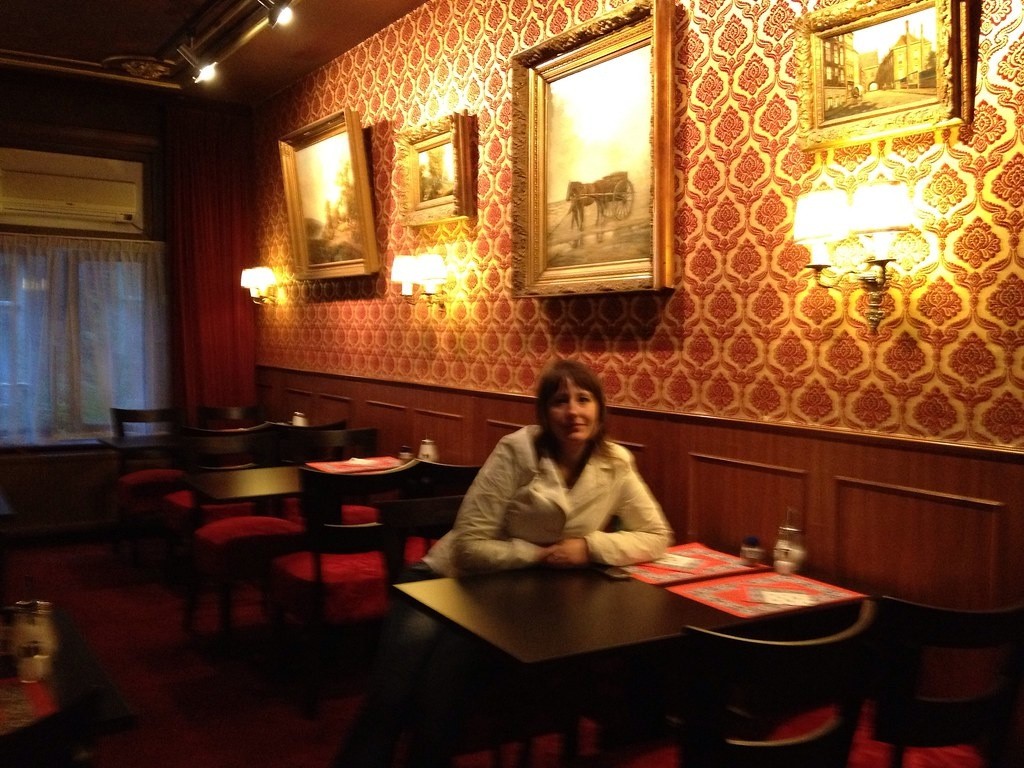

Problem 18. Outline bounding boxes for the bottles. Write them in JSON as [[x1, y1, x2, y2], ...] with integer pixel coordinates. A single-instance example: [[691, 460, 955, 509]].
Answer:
[[419, 439, 439, 462], [293, 411, 305, 427], [741, 536, 761, 566], [399, 446, 413, 465], [15, 598, 57, 683], [774, 525, 799, 574]]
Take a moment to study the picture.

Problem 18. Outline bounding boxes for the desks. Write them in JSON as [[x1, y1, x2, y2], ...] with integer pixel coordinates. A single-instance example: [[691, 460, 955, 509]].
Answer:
[[94, 435, 176, 454], [182, 455, 409, 525], [393, 544, 884, 768], [0, 611, 134, 768]]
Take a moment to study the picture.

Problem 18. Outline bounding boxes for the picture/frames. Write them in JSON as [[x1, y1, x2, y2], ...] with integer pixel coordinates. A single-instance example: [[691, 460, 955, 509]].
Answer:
[[278, 107, 380, 283], [509, 0, 677, 300], [391, 108, 473, 228], [793, 0, 972, 152]]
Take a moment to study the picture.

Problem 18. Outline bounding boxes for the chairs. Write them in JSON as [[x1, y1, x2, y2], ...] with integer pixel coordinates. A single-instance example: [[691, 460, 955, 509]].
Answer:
[[109, 403, 580, 768], [613, 599, 876, 768], [765, 593, 1024, 768]]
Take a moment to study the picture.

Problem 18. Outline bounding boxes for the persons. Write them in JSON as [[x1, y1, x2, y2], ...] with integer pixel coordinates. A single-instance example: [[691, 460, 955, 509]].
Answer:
[[327, 360, 667, 768]]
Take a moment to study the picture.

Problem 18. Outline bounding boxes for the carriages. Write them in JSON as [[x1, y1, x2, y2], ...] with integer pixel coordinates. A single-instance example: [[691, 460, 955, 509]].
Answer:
[[565, 171, 635, 232]]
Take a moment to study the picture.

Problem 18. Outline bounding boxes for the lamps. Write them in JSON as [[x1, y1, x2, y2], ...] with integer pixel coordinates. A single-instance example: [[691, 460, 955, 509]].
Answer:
[[390, 254, 449, 312], [177, 43, 218, 84], [241, 266, 278, 306], [258, 0, 294, 29], [794, 182, 913, 339]]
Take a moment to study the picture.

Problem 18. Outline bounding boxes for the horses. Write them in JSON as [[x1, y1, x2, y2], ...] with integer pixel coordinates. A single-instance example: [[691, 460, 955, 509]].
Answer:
[[566, 180, 612, 231]]
